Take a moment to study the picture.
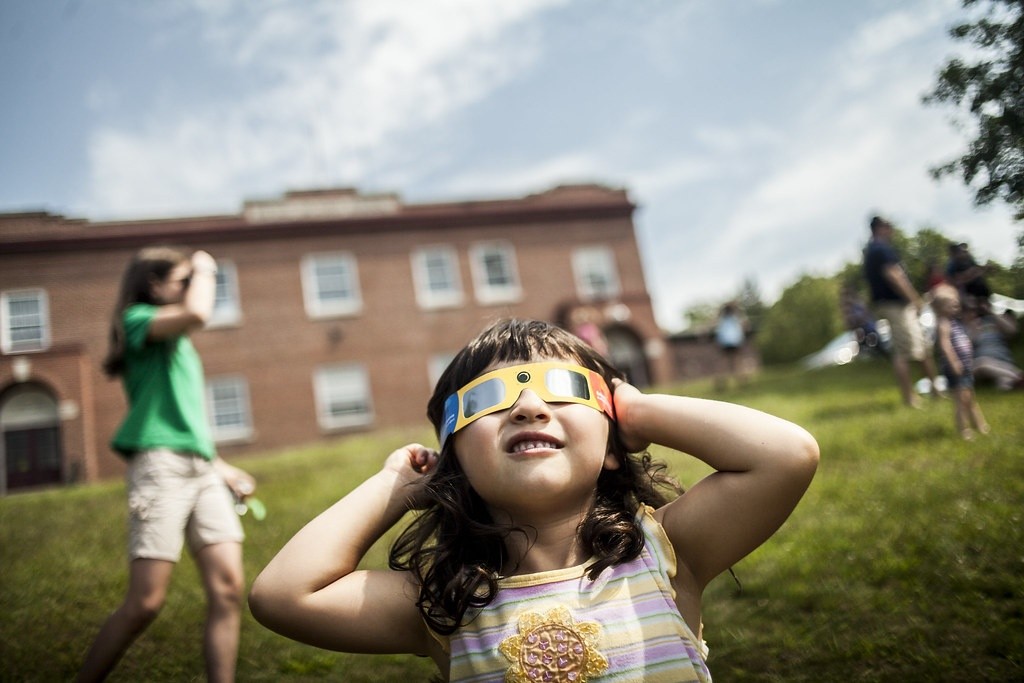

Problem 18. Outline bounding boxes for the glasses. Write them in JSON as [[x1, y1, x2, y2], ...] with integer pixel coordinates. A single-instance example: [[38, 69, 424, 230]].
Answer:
[[155, 278, 189, 288]]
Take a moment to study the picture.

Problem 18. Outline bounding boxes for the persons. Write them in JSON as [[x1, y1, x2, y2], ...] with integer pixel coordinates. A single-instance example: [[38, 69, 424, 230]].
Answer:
[[74, 247, 256, 683], [841, 216, 1024, 441], [570, 308, 609, 369], [715, 302, 754, 391], [248, 316, 821, 683]]
